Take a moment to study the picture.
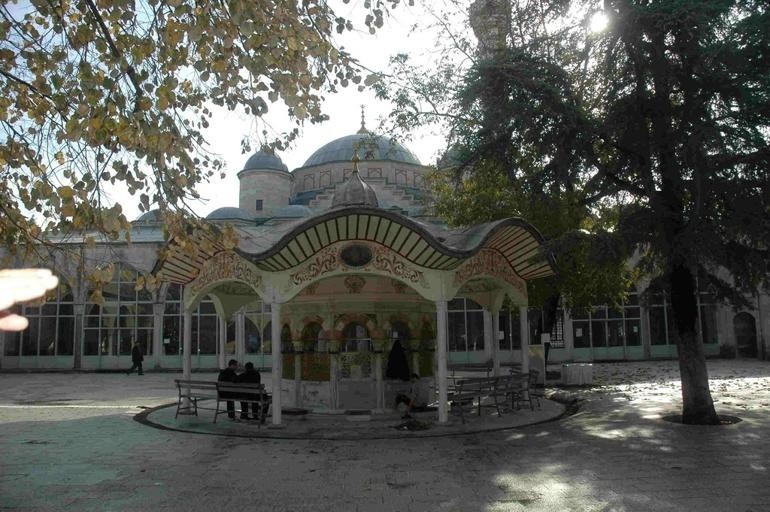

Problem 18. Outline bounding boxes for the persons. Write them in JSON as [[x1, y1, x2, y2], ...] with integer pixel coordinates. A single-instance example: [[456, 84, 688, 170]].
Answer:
[[405, 373, 429, 415], [218, 359, 239, 419], [237, 362, 261, 420], [126, 341, 144, 376]]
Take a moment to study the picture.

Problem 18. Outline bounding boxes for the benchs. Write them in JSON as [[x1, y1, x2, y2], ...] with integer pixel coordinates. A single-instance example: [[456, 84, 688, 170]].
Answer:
[[172, 374, 272, 426], [446, 365, 492, 398], [507, 363, 542, 411], [447, 373, 533, 423]]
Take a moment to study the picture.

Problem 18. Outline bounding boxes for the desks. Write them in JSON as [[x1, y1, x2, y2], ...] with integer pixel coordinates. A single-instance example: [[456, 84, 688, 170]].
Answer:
[[559, 362, 594, 388]]
